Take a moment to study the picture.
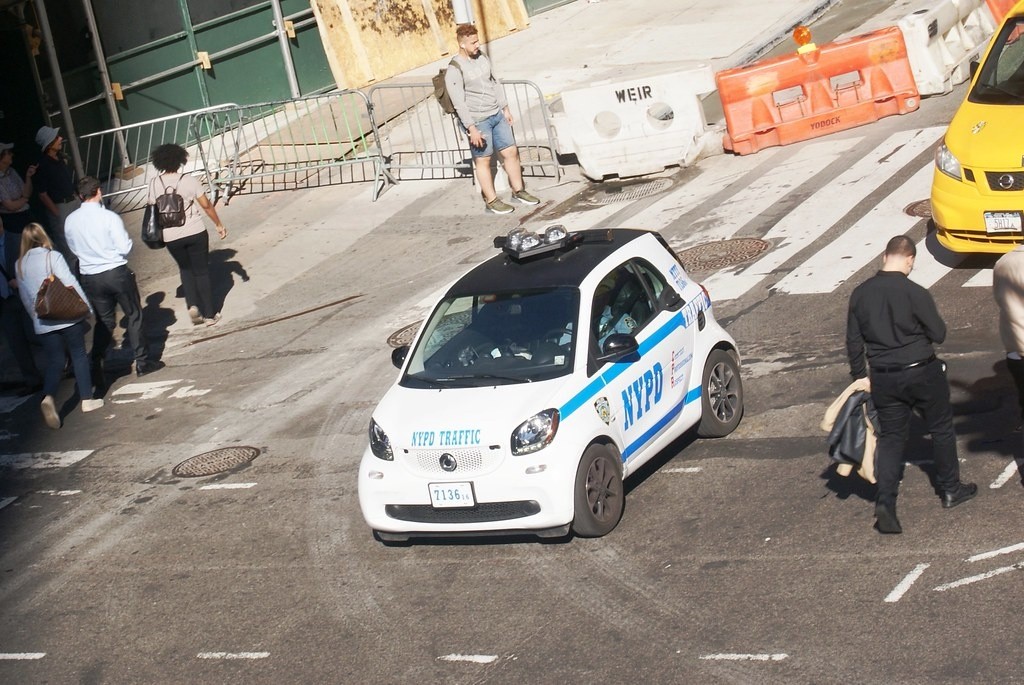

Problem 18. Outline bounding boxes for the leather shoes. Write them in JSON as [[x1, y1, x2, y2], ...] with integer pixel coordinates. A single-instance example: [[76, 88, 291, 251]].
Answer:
[[137, 361, 165, 376], [942, 482, 977, 508], [85, 354, 105, 386], [875, 494, 902, 534]]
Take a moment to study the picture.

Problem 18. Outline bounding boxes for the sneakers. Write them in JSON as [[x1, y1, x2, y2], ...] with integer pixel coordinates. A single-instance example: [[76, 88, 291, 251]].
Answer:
[[512, 191, 540, 205], [485, 199, 514, 214], [82, 398, 105, 411], [41, 395, 61, 428]]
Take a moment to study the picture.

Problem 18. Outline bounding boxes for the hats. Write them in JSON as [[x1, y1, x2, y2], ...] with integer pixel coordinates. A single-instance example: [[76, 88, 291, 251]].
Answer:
[[37, 125, 61, 152], [594, 268, 621, 296], [0, 143, 14, 153]]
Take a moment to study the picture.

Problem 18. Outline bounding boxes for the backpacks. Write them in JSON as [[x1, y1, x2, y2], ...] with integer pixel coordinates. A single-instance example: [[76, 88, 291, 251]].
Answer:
[[432, 51, 492, 113], [155, 172, 194, 229]]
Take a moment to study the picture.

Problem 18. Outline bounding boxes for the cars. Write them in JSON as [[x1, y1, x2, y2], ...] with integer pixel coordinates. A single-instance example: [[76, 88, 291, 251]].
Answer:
[[356, 225, 744, 544], [931, 1, 1024, 255]]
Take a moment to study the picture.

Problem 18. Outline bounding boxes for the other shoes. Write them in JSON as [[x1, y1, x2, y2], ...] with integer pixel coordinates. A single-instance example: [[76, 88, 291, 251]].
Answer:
[[188, 305, 203, 324], [204, 312, 221, 325]]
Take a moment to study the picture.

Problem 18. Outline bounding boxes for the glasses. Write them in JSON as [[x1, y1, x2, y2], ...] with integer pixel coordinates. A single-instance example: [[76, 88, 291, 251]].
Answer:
[[4, 150, 12, 156]]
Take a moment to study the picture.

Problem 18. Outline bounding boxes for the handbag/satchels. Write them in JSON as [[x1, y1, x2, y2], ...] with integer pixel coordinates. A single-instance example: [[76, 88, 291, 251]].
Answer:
[[141, 178, 166, 249], [35, 251, 89, 323]]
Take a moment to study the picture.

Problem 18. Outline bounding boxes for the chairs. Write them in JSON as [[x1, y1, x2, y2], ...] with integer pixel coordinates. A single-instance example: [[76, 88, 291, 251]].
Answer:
[[609, 266, 647, 323]]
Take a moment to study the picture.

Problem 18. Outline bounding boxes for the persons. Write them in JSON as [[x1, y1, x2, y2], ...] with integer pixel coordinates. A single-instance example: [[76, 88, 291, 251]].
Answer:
[[147, 143, 226, 327], [14, 223, 105, 429], [64, 176, 166, 387], [444, 22, 540, 214], [0, 217, 46, 398], [846, 236, 978, 534], [559, 289, 637, 355], [0, 216, 43, 393], [35, 126, 81, 282], [993, 214, 1024, 420], [0, 142, 40, 234]]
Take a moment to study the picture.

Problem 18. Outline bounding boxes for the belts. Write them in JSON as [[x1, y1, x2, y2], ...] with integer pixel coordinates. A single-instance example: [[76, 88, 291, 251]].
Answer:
[[56, 196, 76, 204], [869, 354, 937, 373]]
